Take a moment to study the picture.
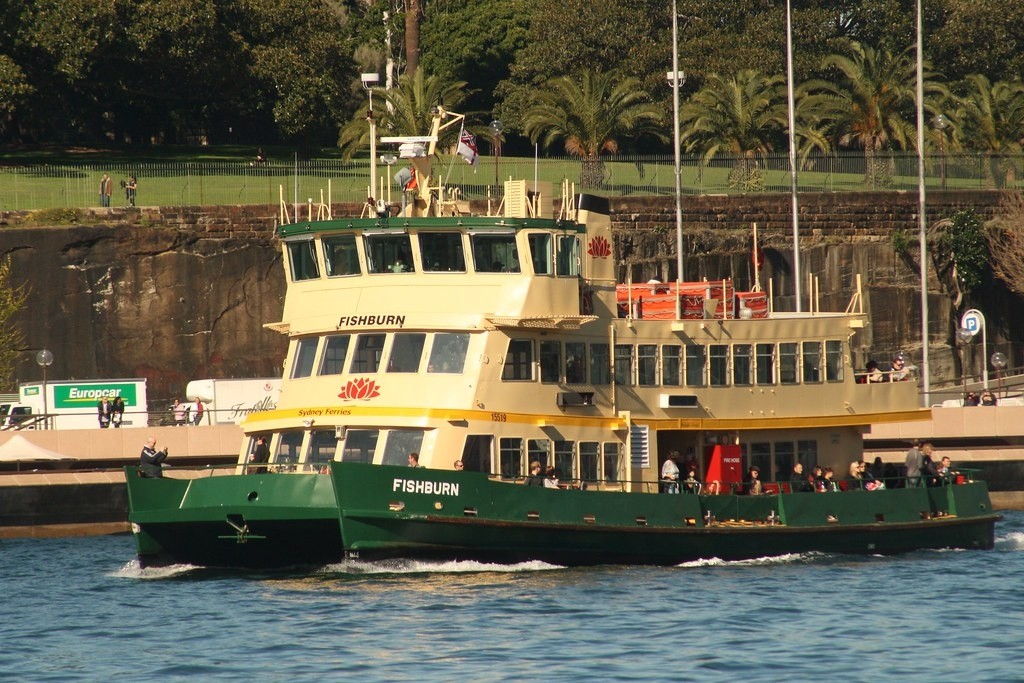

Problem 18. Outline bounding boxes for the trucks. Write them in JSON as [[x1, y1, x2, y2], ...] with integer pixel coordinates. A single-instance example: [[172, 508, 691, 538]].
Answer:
[[0, 377, 149, 431], [176, 377, 281, 428]]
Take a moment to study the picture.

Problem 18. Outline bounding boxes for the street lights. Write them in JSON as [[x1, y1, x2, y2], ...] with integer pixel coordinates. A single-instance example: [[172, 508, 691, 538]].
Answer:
[[934, 114, 949, 191], [990, 352, 1009, 399], [360, 72, 379, 218], [488, 120, 504, 198], [955, 326, 972, 404], [666, 70, 687, 283], [36, 348, 54, 429]]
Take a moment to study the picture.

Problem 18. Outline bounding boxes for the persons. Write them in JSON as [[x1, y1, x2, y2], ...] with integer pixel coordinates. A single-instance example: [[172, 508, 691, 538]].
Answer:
[[906, 439, 960, 486], [97, 396, 124, 429], [889, 359, 917, 382], [964, 389, 997, 406], [140, 438, 168, 477], [126, 176, 137, 206], [408, 453, 419, 467], [847, 457, 901, 490], [99, 173, 113, 207], [868, 361, 883, 382], [661, 450, 701, 493], [792, 464, 837, 491], [428, 364, 435, 372], [172, 397, 205, 427], [250, 435, 270, 474], [743, 466, 774, 494], [454, 460, 464, 470], [524, 462, 587, 490]]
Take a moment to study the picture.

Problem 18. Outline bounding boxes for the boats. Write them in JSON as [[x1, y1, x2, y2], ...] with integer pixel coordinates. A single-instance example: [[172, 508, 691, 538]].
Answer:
[[329, 458, 998, 568], [121, 1, 1024, 580]]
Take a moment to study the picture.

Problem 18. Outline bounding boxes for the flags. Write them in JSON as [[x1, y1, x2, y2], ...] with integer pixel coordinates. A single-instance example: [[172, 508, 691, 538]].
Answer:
[[458, 128, 478, 166]]
